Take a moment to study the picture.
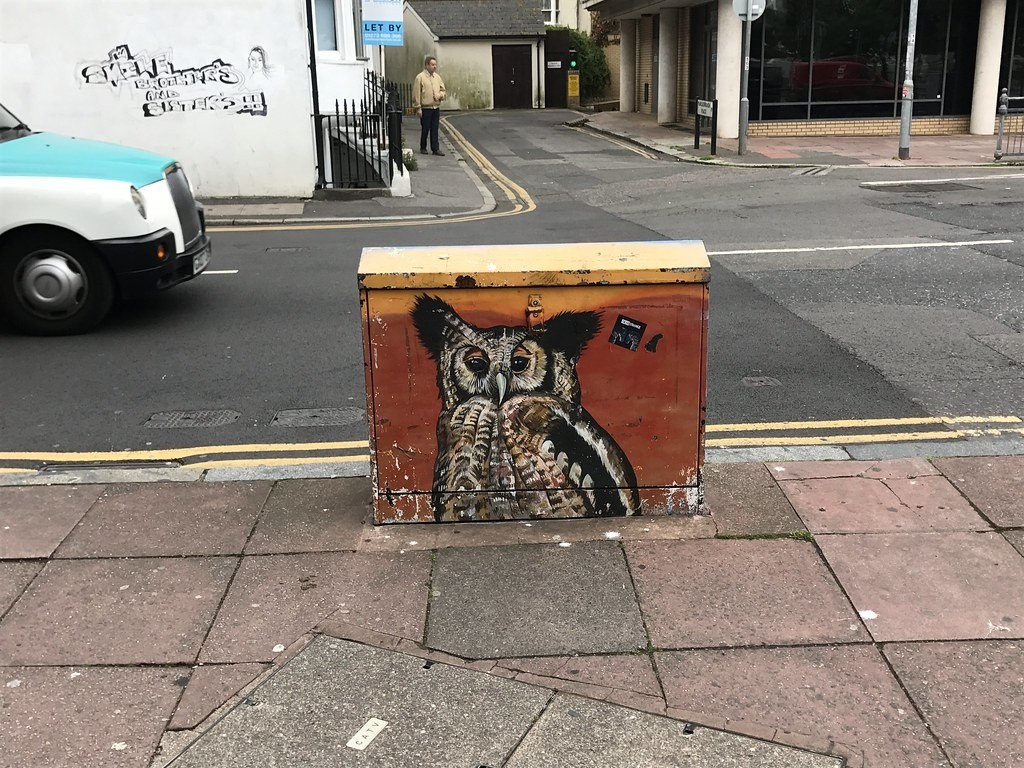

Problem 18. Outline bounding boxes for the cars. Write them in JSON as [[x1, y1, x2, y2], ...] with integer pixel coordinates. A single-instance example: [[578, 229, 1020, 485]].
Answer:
[[767, 57, 901, 90], [747, 60, 791, 121], [0, 104, 212, 335]]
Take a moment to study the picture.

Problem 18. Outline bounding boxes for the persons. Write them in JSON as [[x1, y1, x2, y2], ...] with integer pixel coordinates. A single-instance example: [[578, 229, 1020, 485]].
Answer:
[[412, 57, 446, 156], [825, 47, 838, 58], [870, 51, 889, 81]]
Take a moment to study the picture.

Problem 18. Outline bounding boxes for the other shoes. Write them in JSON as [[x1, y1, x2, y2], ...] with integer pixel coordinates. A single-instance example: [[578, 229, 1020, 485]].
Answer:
[[420, 148, 428, 154], [432, 149, 445, 156]]
